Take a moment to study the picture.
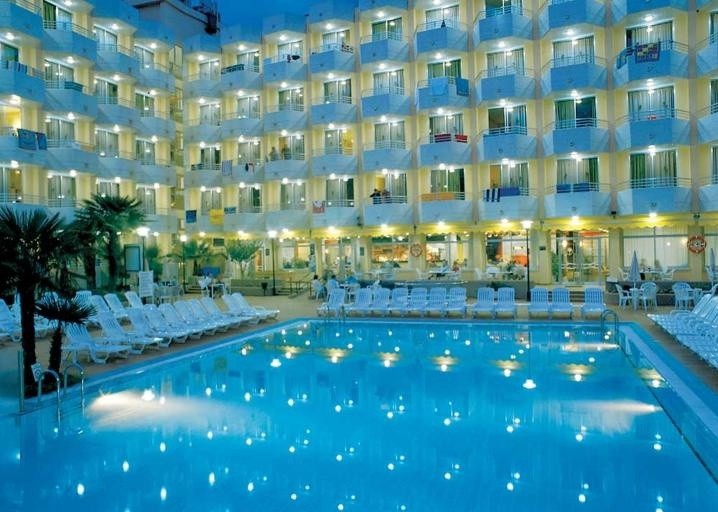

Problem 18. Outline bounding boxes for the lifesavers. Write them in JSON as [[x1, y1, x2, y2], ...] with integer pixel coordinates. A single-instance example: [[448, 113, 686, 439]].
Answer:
[[410, 242, 424, 257], [686, 235, 707, 254]]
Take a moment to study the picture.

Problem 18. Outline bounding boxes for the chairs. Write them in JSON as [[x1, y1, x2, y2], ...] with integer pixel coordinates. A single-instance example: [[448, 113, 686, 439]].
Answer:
[[0, 279, 279, 365], [312, 268, 606, 320], [615, 266, 718, 369]]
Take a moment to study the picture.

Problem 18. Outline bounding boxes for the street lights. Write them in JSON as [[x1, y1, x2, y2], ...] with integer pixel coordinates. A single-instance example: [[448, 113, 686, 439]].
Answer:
[[269, 230, 279, 295], [136, 227, 149, 271], [520, 219, 534, 302], [179, 234, 187, 294]]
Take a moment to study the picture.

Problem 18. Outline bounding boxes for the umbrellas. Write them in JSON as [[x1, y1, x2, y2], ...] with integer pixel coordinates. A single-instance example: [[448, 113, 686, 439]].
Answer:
[[709, 248, 716, 289], [627, 250, 642, 288]]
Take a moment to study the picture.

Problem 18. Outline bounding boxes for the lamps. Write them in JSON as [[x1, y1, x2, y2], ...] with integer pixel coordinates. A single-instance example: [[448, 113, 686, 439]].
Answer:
[[5, 1, 660, 237]]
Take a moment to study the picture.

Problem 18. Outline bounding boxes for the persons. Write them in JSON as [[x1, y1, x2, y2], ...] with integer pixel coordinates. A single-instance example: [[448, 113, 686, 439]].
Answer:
[[146, 278, 161, 306], [435, 261, 448, 279], [268, 147, 278, 161], [280, 143, 291, 160], [200, 272, 217, 299], [313, 275, 325, 300], [328, 274, 337, 292], [369, 188, 381, 204], [381, 188, 391, 203], [452, 261, 461, 274]]
